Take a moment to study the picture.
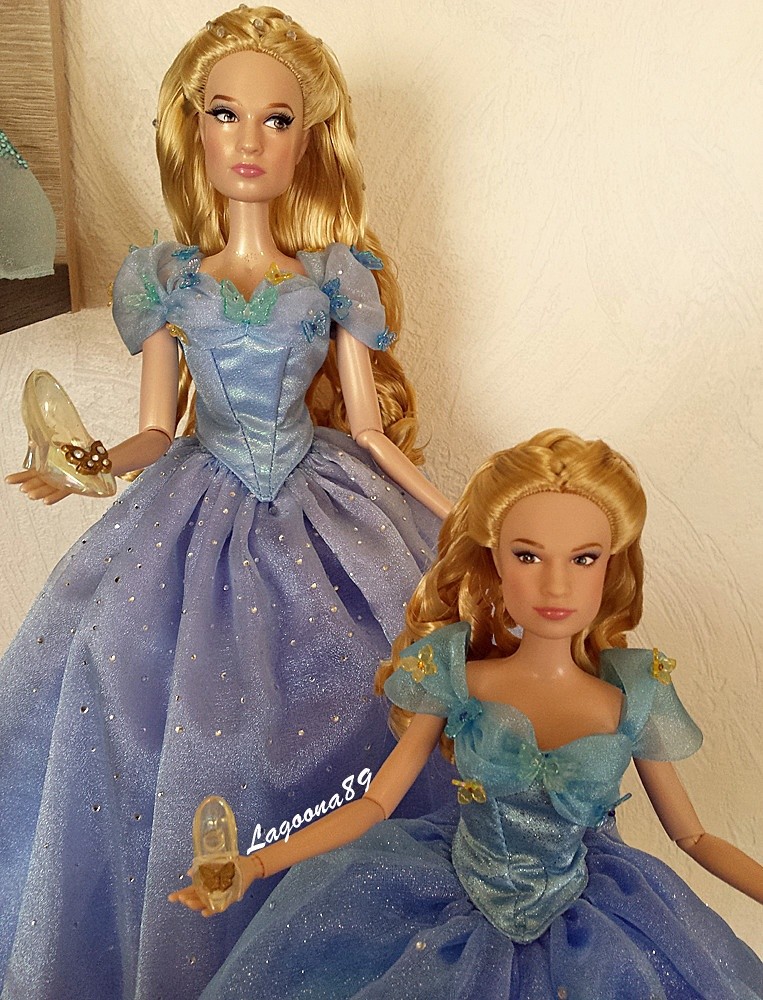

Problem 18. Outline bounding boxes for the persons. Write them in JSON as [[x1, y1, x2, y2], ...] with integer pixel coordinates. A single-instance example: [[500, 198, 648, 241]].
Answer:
[[166, 427, 762, 1000], [1, 5, 458, 1000]]
